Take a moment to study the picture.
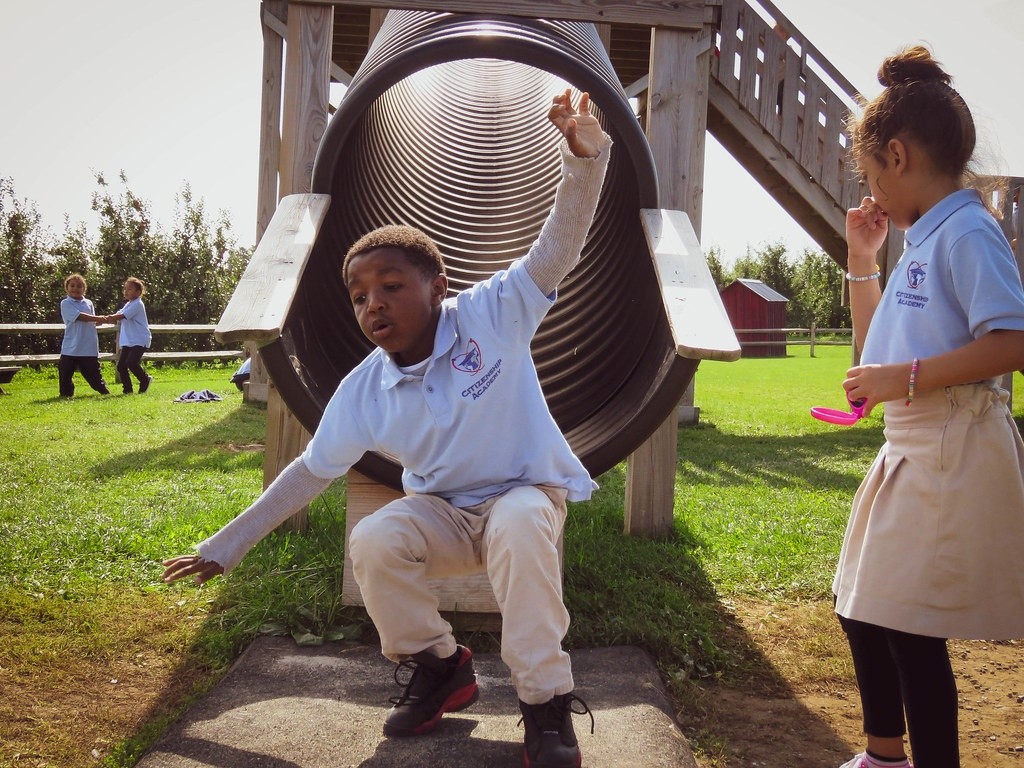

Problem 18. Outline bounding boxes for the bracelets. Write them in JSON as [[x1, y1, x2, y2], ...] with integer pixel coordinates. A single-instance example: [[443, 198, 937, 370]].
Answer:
[[846, 264, 881, 281], [907, 355, 919, 408]]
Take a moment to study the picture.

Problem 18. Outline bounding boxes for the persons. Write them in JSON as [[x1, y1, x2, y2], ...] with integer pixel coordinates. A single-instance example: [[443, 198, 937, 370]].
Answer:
[[161, 91, 612, 767], [833, 47, 1023, 768], [229, 357, 252, 391], [59, 275, 152, 397]]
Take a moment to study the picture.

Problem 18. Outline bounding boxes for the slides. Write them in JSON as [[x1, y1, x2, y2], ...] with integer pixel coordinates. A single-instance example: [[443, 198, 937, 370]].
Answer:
[[259, 8, 708, 497]]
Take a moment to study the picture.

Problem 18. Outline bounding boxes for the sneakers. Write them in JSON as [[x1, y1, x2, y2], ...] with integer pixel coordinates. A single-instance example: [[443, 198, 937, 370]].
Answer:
[[517, 691, 594, 768], [838, 753, 912, 768], [137, 376, 153, 394], [382, 644, 480, 738]]
